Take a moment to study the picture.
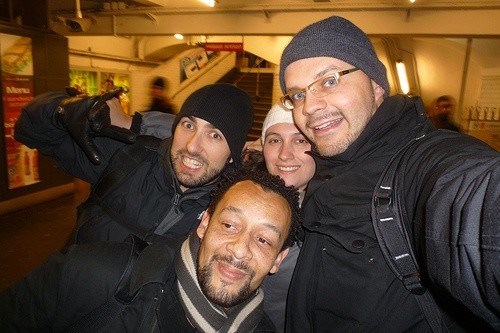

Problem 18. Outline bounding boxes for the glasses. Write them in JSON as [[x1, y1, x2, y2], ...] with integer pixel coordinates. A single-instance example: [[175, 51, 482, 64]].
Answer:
[[280, 67, 361, 110]]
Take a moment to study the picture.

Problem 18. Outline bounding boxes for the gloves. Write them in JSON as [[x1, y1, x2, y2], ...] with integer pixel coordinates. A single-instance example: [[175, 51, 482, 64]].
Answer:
[[50, 86, 137, 166]]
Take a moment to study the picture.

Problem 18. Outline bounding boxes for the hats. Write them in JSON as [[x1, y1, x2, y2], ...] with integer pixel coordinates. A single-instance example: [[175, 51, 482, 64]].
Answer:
[[172, 83, 255, 171], [261, 102, 295, 146], [278, 15, 391, 100]]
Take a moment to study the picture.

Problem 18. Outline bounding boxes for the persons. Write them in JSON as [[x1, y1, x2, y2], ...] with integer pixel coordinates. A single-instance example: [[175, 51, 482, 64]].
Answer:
[[105, 97, 317, 333], [244, 16, 500, 333], [429, 96, 459, 131], [1, 163, 304, 333], [148, 78, 175, 113], [15, 82, 254, 244]]
